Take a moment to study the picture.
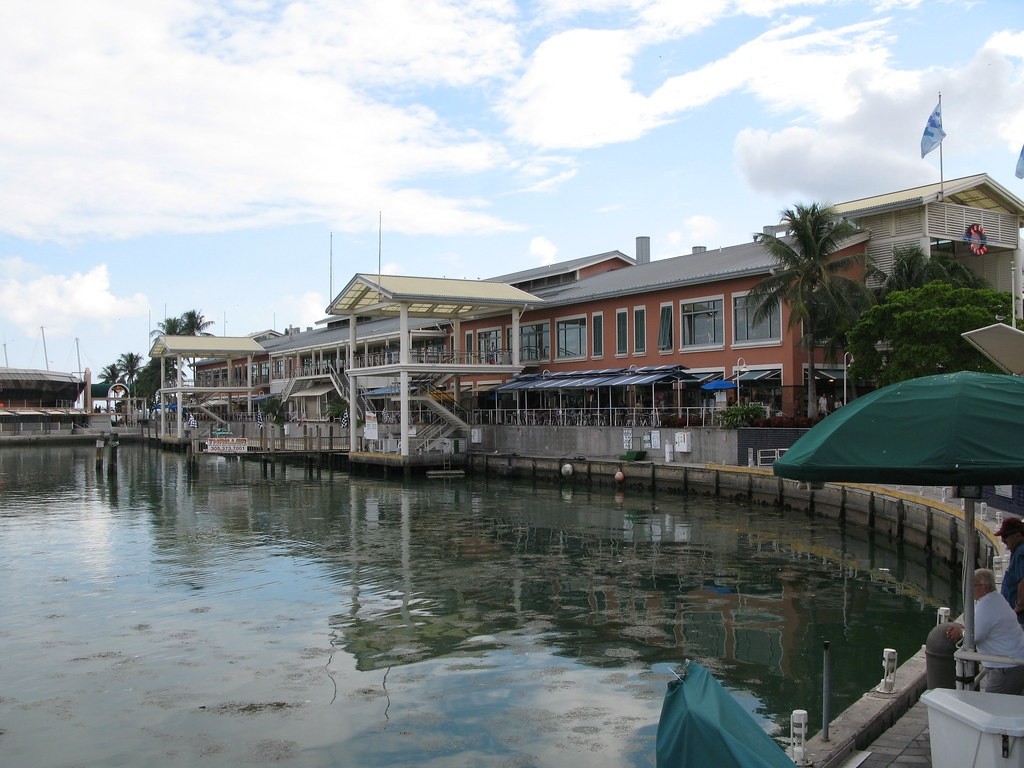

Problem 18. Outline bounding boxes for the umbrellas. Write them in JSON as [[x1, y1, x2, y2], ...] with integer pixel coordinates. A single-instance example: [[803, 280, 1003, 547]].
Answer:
[[701, 378, 738, 390], [770, 369, 1024, 652]]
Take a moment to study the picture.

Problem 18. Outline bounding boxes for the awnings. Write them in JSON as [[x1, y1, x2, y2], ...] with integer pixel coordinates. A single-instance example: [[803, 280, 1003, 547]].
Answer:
[[488, 365, 700, 389], [360, 386, 418, 395], [723, 370, 780, 379], [249, 392, 277, 401], [805, 369, 849, 380]]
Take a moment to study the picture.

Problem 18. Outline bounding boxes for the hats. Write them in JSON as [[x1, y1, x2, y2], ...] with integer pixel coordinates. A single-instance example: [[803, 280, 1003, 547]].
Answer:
[[994, 517, 1021, 536]]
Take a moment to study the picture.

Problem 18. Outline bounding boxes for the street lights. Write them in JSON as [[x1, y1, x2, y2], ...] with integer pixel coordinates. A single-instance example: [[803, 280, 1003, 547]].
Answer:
[[737, 357, 747, 407], [844, 352, 855, 407]]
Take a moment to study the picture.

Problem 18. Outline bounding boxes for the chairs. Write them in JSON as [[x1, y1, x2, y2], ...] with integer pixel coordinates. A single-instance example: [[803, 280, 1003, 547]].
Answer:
[[507, 414, 700, 426]]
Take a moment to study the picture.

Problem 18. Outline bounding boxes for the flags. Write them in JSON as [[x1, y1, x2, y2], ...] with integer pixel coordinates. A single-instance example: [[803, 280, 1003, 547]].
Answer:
[[920, 102, 946, 159], [1014, 144, 1024, 179]]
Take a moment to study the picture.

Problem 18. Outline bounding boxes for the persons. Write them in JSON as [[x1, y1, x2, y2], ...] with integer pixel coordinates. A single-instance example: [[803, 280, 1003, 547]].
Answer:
[[945, 516, 1024, 695]]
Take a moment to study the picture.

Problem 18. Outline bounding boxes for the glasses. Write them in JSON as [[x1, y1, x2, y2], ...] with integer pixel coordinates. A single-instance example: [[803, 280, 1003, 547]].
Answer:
[[1002, 530, 1017, 539]]
